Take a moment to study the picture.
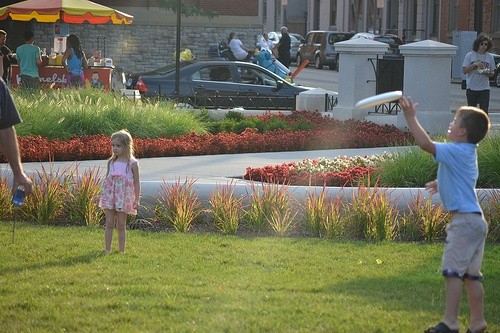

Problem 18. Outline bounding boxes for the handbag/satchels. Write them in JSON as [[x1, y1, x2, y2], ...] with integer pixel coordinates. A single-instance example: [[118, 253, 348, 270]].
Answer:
[[73, 76, 83, 87]]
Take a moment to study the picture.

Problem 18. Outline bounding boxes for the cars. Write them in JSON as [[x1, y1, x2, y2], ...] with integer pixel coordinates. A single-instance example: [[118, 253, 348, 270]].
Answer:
[[352, 32, 404, 47], [267, 31, 304, 58], [489, 51, 500, 90], [126, 58, 314, 110]]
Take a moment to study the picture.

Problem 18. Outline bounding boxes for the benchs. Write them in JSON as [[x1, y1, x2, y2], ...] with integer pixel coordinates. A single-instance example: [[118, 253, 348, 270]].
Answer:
[[210, 67, 230, 81]]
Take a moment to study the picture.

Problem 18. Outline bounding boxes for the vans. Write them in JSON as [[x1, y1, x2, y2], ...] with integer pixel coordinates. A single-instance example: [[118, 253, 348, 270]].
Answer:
[[296, 31, 354, 70]]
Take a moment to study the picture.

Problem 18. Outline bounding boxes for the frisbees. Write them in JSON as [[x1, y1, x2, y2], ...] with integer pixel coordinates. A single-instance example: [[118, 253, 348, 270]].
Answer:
[[356, 90, 403, 111]]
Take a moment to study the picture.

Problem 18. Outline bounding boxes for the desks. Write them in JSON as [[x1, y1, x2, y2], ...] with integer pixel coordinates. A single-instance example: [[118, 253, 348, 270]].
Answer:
[[11, 64, 113, 90]]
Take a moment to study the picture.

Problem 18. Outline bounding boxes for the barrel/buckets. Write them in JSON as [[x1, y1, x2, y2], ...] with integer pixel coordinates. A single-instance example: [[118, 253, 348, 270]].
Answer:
[[49, 55, 56, 65], [56, 55, 63, 65], [42, 56, 49, 66]]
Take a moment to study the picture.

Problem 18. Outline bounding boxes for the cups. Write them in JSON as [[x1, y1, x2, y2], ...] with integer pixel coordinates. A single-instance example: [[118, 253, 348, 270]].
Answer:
[[12, 189, 25, 206]]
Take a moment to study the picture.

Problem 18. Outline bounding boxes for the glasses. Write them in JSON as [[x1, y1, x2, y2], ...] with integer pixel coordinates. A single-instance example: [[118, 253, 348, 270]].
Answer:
[[481, 43, 488, 46]]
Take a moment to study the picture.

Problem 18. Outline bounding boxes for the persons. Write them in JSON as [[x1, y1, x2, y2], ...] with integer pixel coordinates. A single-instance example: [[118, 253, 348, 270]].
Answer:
[[256, 32, 276, 52], [0, 30, 16, 83], [399, 95, 490, 333], [16, 30, 43, 89], [271, 27, 291, 68], [61, 34, 87, 89], [90, 72, 103, 90], [228, 32, 254, 61], [91, 130, 140, 253], [462, 34, 496, 115], [0, 77, 35, 196]]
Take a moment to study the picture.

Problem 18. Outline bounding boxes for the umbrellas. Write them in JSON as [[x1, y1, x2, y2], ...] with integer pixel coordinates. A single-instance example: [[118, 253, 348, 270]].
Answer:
[[0, 0, 134, 25]]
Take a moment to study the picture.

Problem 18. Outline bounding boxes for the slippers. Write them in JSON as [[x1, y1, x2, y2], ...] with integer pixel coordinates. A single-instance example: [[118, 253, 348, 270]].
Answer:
[[424, 321, 459, 333], [466, 327, 489, 333]]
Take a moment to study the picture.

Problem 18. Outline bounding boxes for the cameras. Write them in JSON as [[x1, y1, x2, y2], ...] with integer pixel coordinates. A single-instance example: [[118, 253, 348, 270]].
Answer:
[[11, 190, 25, 207]]
